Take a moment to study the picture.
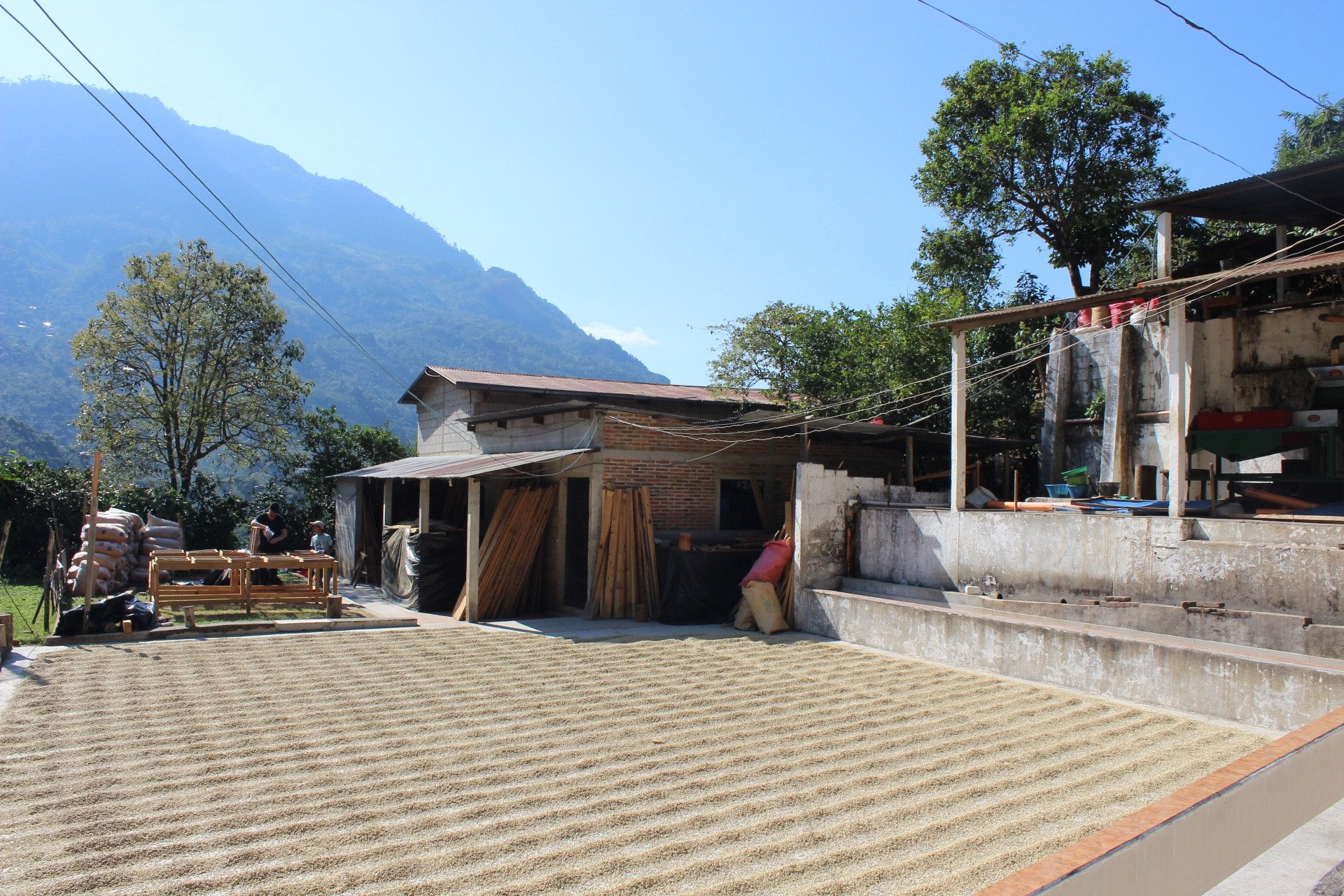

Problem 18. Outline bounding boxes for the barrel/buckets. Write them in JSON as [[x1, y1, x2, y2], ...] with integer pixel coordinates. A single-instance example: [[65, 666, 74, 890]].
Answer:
[[1064, 476, 1089, 485], [1066, 485, 1091, 498], [1095, 482, 1121, 497]]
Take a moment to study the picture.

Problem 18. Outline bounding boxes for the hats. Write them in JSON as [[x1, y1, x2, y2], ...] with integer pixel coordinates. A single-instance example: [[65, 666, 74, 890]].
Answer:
[[309, 521, 325, 528], [272, 503, 280, 512]]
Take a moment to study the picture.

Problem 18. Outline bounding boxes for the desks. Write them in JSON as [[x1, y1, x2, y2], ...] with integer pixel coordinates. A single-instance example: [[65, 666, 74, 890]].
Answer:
[[656, 541, 767, 624], [1201, 294, 1250, 321]]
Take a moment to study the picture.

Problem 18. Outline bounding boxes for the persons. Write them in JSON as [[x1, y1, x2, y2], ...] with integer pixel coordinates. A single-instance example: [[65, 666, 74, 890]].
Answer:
[[251, 502, 287, 585], [309, 520, 334, 593]]
